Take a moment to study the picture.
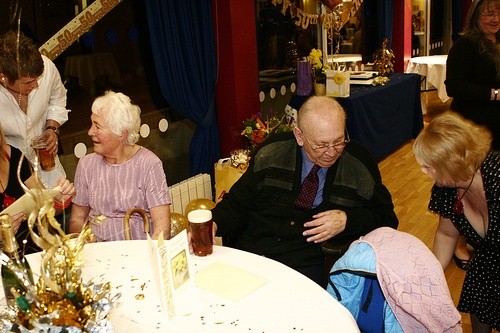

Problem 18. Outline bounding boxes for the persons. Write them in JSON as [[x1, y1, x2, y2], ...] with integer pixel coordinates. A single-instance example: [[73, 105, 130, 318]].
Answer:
[[446, 0, 500, 125], [187, 96, 399, 291], [0, 32, 69, 190], [413, 114, 500, 333], [68, 92, 171, 242], [0, 121, 77, 256]]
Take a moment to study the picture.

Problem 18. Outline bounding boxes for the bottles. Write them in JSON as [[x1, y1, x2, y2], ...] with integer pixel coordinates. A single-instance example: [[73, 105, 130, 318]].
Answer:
[[0, 213, 34, 311]]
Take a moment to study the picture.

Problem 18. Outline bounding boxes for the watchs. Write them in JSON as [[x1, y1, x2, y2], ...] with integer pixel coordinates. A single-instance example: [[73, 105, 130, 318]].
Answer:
[[494, 89, 498, 100]]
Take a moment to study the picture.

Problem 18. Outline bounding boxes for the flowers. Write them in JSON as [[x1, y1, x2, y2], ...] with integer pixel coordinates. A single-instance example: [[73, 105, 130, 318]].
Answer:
[[240, 107, 293, 155], [308, 48, 327, 84]]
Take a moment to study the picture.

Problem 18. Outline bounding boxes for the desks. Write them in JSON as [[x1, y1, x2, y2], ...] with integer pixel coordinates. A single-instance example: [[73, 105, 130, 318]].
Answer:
[[289, 73, 424, 163], [406, 55, 453, 103], [23, 239, 361, 333]]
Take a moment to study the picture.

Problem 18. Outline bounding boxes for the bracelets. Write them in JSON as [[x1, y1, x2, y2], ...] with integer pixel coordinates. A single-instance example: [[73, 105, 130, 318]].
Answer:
[[55, 199, 71, 208], [46, 125, 59, 136]]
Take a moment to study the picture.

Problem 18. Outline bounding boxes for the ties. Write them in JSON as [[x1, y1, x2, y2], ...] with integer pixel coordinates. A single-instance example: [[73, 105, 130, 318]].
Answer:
[[292, 163, 321, 217]]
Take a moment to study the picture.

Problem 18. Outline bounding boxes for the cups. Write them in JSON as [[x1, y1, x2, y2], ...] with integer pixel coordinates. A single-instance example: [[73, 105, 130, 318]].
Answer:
[[61, 233, 86, 267], [31, 135, 56, 172], [188, 210, 213, 257]]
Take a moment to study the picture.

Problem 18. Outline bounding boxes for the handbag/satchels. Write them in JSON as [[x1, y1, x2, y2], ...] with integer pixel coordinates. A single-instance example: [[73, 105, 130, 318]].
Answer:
[[325, 61, 351, 98], [214, 157, 246, 205]]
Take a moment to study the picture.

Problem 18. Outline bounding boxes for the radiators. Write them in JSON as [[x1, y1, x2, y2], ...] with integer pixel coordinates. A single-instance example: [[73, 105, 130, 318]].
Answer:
[[168, 173, 213, 215]]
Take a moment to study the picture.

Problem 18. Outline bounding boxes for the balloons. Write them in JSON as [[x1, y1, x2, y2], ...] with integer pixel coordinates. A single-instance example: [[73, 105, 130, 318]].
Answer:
[[185, 198, 216, 217], [170, 212, 188, 237]]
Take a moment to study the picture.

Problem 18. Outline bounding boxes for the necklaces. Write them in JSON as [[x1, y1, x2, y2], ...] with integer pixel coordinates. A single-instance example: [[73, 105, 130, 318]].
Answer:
[[125, 145, 134, 160], [0, 180, 16, 208], [18, 94, 21, 107], [454, 171, 476, 213]]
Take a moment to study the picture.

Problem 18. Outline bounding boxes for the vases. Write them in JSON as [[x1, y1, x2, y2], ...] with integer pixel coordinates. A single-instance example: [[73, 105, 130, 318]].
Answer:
[[313, 83, 326, 96]]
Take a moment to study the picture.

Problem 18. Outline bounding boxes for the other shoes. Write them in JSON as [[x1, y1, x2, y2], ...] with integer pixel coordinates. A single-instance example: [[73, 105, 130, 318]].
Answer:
[[453, 253, 472, 270]]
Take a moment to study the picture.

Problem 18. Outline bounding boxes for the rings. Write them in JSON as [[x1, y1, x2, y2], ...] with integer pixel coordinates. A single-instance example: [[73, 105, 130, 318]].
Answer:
[[56, 142, 58, 144]]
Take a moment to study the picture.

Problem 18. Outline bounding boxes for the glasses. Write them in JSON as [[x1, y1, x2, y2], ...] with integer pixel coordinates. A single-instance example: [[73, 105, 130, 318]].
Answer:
[[479, 12, 500, 20], [299, 125, 350, 153]]
